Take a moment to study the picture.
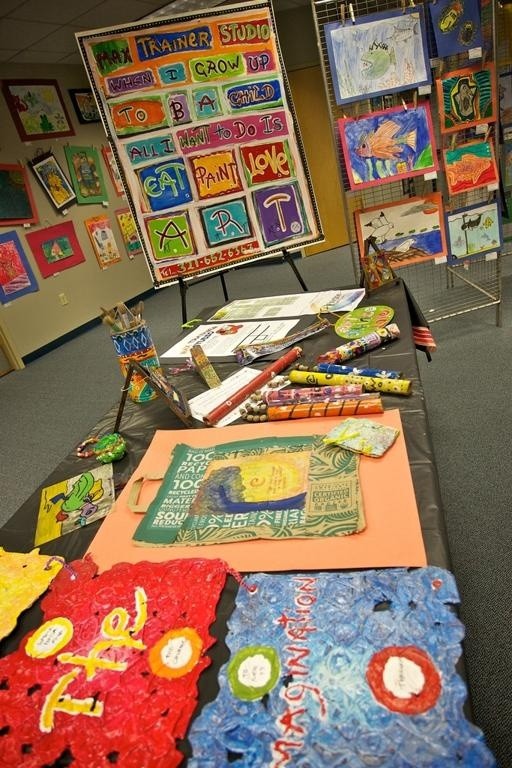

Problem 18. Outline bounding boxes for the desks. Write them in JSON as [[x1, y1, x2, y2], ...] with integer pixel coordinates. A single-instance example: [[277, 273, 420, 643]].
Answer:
[[0, 279, 475, 768]]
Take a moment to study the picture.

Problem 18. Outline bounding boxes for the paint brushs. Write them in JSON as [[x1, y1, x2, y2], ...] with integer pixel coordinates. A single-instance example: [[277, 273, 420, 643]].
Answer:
[[100, 301, 144, 330]]
